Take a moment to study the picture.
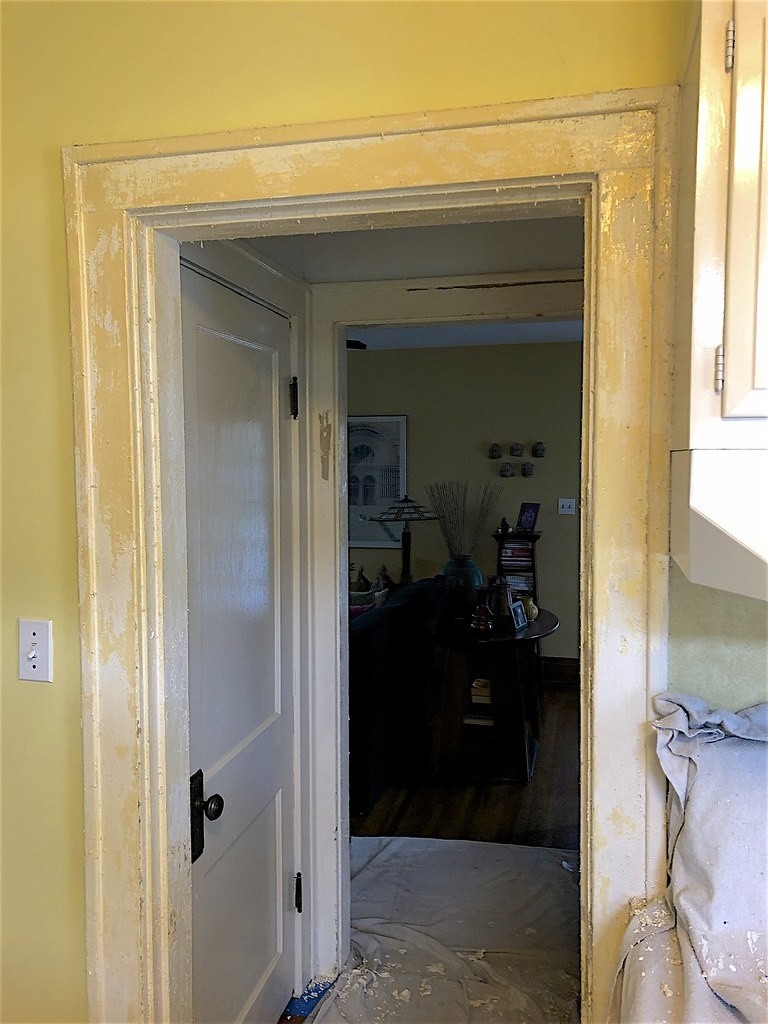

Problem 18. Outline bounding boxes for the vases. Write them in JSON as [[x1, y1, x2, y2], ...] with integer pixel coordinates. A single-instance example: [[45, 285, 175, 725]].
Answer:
[[470, 587, 493, 630], [441, 556, 484, 605]]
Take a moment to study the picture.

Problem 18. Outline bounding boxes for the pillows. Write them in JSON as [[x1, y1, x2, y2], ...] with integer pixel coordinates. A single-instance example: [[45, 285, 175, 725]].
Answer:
[[350, 588, 389, 619]]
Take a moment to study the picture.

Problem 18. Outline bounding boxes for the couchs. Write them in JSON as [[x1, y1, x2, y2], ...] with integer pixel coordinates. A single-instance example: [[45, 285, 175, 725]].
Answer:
[[349, 576, 453, 823]]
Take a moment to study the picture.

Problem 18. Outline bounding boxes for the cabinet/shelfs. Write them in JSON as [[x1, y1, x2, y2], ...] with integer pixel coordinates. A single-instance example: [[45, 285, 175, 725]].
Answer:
[[490, 533, 540, 606], [675, 0, 768, 451]]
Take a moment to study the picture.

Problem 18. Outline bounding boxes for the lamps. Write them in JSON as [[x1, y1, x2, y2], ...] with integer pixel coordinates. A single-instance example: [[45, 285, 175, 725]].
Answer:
[[371, 494, 445, 587]]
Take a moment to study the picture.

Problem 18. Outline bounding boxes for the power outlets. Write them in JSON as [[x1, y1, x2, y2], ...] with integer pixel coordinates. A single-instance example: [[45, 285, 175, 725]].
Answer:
[[558, 499, 576, 514]]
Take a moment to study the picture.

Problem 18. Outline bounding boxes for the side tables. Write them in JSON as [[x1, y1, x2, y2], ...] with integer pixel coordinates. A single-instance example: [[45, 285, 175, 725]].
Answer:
[[419, 602, 561, 783]]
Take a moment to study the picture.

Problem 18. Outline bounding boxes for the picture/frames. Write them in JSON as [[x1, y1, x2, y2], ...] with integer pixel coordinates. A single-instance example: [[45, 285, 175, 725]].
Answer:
[[347, 415, 406, 549], [518, 502, 540, 531]]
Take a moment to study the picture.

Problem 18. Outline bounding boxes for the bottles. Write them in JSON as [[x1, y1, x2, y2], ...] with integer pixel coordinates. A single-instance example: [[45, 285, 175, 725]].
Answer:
[[516, 596, 538, 622]]
